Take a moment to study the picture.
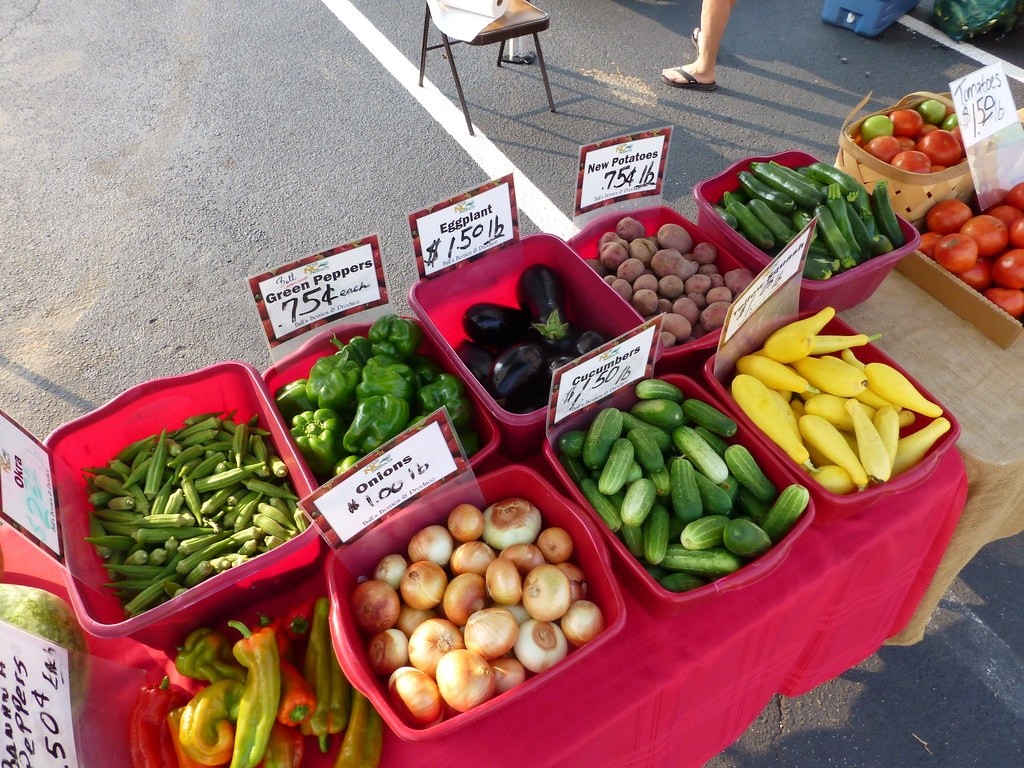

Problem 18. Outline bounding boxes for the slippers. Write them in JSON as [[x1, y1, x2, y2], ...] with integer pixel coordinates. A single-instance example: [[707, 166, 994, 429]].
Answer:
[[692, 30, 718, 64], [660, 66, 716, 90]]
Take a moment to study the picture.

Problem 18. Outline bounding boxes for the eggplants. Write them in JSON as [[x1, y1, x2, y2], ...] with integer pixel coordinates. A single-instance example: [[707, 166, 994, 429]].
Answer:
[[454, 265, 609, 412]]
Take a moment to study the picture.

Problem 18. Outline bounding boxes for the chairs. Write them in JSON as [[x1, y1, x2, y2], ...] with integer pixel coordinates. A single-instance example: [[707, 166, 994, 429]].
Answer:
[[419, 0, 556, 136]]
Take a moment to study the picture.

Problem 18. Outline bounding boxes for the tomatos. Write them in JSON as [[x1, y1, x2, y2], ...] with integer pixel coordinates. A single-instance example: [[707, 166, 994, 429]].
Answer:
[[858, 99, 966, 175], [920, 181, 1024, 317]]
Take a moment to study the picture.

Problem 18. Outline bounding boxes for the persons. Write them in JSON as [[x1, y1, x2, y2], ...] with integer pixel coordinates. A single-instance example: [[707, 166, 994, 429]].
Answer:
[[660, 0, 735, 91]]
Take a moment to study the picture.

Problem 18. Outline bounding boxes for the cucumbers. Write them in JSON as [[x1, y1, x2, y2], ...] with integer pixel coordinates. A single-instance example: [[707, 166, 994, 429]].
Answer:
[[560, 378, 809, 592]]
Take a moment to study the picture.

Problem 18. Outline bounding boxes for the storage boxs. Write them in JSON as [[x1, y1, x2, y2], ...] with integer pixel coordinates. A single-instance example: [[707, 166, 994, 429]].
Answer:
[[42, 360, 323, 652], [406, 232, 664, 460], [261, 316, 502, 496], [703, 308, 961, 528], [566, 206, 757, 374], [691, 150, 921, 313], [322, 464, 627, 743], [894, 219, 1024, 351], [543, 373, 815, 620]]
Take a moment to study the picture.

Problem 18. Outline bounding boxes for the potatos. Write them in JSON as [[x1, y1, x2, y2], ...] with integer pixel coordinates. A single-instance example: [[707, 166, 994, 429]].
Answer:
[[585, 217, 755, 351]]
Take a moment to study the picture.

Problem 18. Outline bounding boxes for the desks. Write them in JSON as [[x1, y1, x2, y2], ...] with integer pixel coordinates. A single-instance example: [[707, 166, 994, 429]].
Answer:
[[835, 268, 1024, 646], [0, 446, 971, 768]]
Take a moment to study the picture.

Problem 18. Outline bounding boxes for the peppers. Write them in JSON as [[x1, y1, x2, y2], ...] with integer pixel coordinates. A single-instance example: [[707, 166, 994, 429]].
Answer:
[[130, 598, 382, 768], [276, 314, 482, 482]]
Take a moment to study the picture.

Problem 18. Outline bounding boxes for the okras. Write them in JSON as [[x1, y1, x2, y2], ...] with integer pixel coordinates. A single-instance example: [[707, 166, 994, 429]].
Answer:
[[79, 410, 307, 621]]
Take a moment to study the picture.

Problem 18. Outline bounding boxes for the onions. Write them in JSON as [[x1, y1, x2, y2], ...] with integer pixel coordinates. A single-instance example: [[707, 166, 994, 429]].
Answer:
[[350, 498, 606, 728]]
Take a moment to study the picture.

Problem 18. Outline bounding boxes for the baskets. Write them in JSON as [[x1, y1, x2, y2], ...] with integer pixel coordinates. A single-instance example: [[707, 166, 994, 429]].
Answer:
[[833, 91, 974, 231]]
